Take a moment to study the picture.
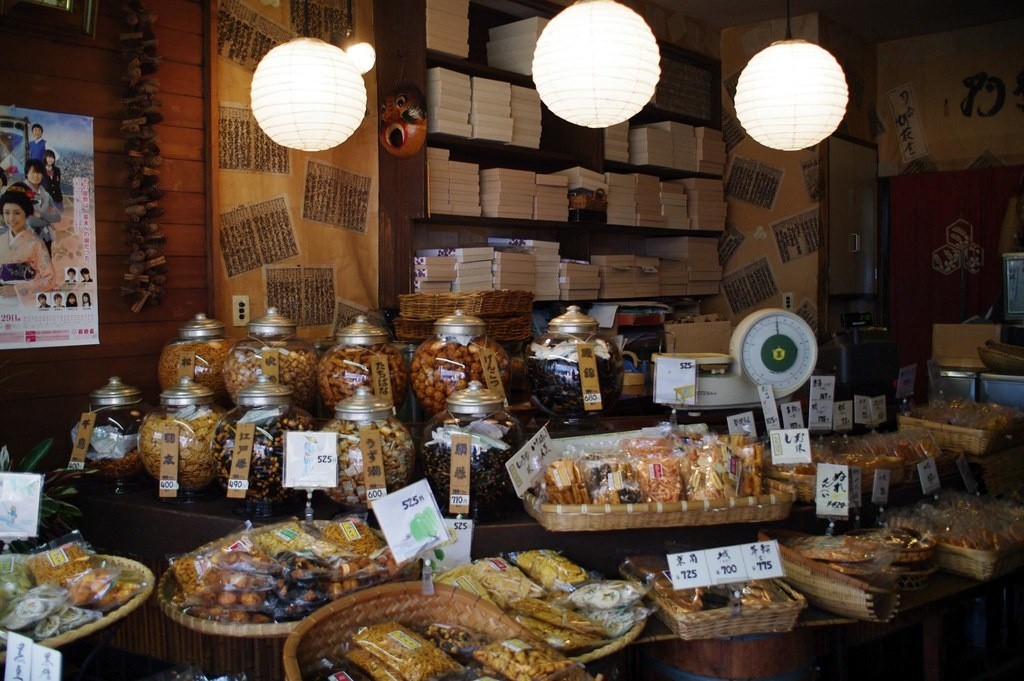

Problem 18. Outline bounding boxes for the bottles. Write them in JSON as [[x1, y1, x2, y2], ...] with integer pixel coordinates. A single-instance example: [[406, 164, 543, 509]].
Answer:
[[84, 304, 624, 525]]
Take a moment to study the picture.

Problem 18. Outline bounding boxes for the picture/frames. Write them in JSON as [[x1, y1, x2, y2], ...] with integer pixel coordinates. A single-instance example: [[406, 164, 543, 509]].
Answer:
[[0, 0, 97, 43]]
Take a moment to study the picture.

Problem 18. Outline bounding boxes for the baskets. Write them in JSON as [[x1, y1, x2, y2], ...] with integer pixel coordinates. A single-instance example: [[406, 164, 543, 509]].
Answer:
[[985, 339, 1024, 358], [0, 550, 155, 665], [392, 316, 533, 343], [154, 401, 1024, 681], [398, 287, 535, 321], [977, 346, 1024, 373]]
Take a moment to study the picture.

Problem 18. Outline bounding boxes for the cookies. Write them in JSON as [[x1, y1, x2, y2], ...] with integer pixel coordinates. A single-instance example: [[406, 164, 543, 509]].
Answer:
[[542, 460, 591, 505], [819, 402, 1024, 552]]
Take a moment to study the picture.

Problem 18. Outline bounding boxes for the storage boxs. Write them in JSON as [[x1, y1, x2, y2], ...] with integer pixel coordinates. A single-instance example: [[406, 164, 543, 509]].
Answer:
[[616, 312, 661, 327], [663, 314, 732, 354], [932, 323, 1002, 372], [918, 367, 978, 404], [978, 373, 1024, 409]]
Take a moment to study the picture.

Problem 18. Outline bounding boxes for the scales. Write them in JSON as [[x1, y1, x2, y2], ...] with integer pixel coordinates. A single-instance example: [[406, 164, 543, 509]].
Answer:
[[653, 307, 818, 408]]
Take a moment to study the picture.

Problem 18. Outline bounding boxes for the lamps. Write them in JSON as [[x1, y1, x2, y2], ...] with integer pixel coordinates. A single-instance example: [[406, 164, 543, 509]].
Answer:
[[530, 0, 663, 131], [733, 0, 850, 152], [248, 0, 368, 153]]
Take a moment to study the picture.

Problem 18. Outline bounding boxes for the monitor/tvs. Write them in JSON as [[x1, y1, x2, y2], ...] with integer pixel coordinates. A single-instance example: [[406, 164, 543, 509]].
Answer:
[[1002, 252, 1024, 320]]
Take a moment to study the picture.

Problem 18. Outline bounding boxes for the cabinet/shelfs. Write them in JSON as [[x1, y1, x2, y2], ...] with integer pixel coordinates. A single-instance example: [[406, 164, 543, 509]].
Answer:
[[889, 163, 1024, 406], [371, 0, 724, 319], [828, 132, 880, 303], [69, 464, 1024, 681]]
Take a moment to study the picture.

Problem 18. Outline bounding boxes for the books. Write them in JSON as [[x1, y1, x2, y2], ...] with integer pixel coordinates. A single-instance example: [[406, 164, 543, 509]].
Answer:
[[411, 1, 727, 302]]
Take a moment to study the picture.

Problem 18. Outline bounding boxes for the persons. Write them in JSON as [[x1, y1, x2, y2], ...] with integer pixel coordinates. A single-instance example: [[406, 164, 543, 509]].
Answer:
[[37, 294, 50, 308], [51, 293, 66, 308], [0, 182, 56, 298], [66, 293, 77, 307], [17, 159, 61, 259], [66, 268, 78, 282], [41, 150, 63, 210], [81, 293, 92, 308], [80, 268, 93, 282], [26, 123, 61, 164]]
